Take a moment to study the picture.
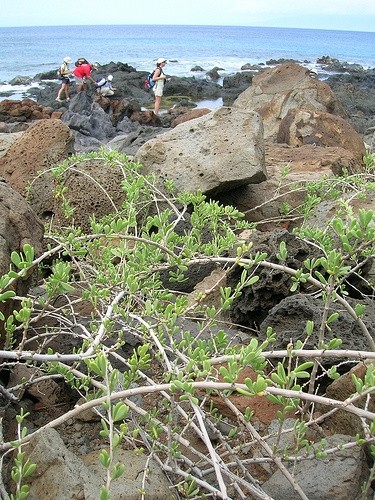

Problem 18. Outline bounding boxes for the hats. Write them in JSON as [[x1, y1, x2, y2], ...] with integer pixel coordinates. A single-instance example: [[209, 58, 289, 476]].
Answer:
[[64, 57, 73, 64], [158, 58, 167, 64], [108, 75, 113, 81], [93, 62, 100, 71]]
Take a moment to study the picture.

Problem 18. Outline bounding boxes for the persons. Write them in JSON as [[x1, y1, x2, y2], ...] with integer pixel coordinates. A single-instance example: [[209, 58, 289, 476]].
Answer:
[[56, 57, 118, 103], [152, 58, 167, 115]]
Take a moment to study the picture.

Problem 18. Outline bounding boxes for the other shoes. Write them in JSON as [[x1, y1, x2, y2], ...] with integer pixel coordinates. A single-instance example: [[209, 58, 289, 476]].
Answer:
[[66, 99, 70, 102], [55, 97, 62, 102], [102, 93, 106, 97]]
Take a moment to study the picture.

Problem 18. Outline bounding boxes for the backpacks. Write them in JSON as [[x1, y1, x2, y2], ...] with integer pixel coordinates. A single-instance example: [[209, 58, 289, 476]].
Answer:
[[145, 68, 161, 89], [95, 77, 107, 89]]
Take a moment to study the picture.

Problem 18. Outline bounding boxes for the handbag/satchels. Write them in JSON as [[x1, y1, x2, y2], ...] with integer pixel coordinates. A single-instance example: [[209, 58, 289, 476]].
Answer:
[[56, 66, 63, 80], [75, 58, 89, 67]]
